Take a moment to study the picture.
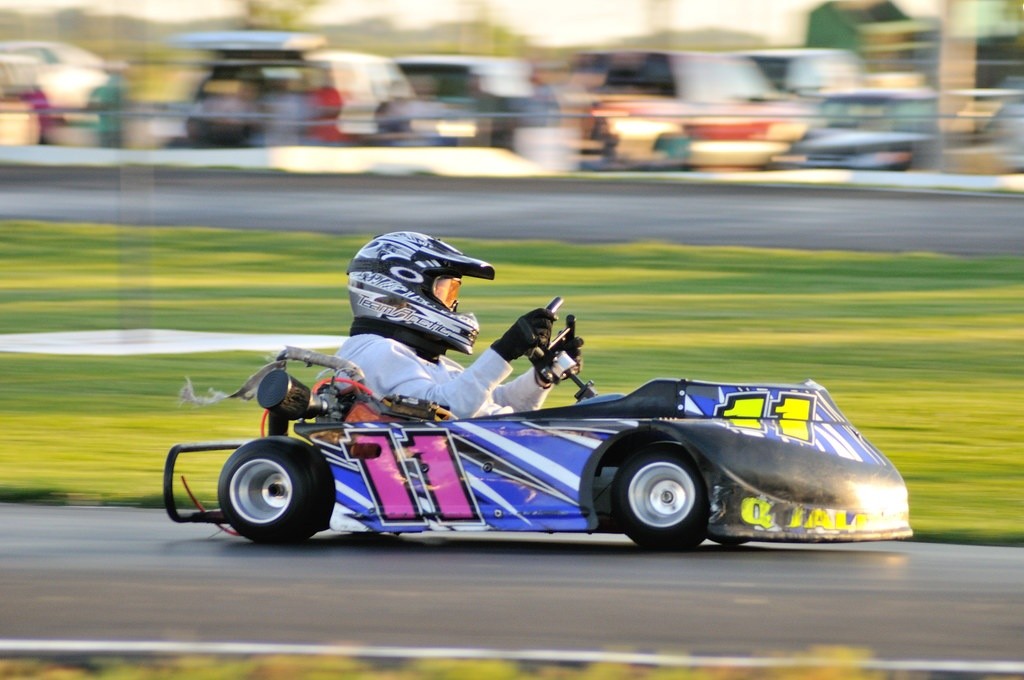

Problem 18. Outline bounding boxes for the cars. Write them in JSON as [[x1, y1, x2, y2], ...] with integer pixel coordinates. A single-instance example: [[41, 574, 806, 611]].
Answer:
[[1, 36, 129, 151], [147, 24, 1024, 175]]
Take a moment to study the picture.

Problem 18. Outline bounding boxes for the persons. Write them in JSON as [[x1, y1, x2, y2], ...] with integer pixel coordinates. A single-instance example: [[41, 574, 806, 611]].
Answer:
[[17, 81, 68, 145], [201, 67, 562, 148], [86, 65, 136, 149], [334, 231, 628, 419]]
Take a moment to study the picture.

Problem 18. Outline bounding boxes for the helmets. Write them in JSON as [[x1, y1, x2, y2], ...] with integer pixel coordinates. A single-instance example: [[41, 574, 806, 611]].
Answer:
[[348, 232, 495, 356]]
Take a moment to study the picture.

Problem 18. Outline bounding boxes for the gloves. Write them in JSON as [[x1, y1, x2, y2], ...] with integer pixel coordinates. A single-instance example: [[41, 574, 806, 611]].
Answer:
[[528, 329, 582, 382], [493, 308, 559, 362]]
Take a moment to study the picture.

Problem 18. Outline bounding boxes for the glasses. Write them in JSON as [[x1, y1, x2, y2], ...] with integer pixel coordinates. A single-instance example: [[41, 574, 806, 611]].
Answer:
[[434, 276, 462, 310]]
